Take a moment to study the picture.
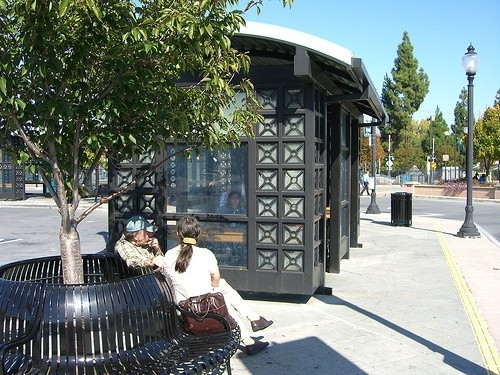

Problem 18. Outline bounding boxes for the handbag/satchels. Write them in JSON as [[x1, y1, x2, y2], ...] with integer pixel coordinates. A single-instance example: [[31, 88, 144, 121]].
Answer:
[[179, 292, 237, 334]]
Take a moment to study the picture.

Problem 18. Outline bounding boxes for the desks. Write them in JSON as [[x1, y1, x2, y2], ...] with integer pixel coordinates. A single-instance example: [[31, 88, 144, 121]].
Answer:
[[168, 226, 244, 244]]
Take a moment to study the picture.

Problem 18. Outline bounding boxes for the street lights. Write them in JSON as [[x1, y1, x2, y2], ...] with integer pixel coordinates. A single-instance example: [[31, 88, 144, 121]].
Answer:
[[456, 41, 481, 238]]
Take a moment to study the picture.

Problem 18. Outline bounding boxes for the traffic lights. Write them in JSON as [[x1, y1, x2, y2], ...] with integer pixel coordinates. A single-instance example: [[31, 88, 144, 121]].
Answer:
[[390, 157, 394, 161], [383, 157, 389, 161]]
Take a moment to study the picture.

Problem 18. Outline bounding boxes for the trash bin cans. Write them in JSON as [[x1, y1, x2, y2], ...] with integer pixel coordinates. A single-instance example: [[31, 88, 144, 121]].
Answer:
[[391, 192, 412, 227]]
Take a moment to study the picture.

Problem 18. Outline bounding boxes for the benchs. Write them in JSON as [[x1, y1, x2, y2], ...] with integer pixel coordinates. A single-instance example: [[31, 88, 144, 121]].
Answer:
[[0, 254, 242, 375], [93, 184, 108, 202]]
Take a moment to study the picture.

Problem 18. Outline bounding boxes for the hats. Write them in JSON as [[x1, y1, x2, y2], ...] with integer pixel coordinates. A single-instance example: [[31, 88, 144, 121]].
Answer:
[[125, 215, 158, 232]]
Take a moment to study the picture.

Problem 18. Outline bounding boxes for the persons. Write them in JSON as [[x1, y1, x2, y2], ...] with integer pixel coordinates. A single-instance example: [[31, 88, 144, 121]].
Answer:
[[358, 170, 371, 196], [220, 192, 249, 230], [161, 217, 273, 356], [473, 172, 486, 182], [115, 215, 164, 269]]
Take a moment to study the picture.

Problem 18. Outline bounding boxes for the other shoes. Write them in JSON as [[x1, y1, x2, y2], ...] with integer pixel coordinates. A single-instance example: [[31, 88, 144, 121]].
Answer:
[[245, 340, 270, 355], [251, 316, 273, 332]]
